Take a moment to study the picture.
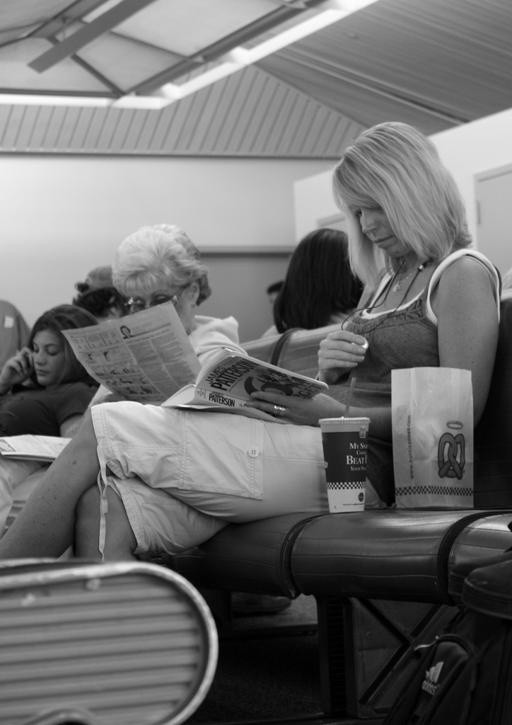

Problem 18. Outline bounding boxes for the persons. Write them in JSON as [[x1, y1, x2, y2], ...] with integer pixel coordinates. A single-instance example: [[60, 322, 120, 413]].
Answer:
[[0, 224, 365, 615], [2, 122, 503, 567]]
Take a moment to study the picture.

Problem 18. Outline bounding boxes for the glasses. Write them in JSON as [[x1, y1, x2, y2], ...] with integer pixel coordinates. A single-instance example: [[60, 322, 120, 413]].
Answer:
[[123, 283, 189, 314]]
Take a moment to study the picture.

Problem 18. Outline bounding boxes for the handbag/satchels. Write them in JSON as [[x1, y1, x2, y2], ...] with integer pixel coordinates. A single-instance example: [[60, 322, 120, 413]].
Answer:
[[384, 610, 511, 725]]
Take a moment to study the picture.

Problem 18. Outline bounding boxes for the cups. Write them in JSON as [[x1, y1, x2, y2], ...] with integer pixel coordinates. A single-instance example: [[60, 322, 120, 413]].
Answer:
[[319, 417, 372, 513]]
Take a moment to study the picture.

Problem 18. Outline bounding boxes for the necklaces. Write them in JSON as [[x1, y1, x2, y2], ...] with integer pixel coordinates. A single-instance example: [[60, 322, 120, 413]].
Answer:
[[393, 270, 414, 291]]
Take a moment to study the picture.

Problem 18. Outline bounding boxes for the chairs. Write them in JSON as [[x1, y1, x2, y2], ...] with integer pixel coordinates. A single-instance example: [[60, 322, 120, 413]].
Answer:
[[193, 293, 508, 724]]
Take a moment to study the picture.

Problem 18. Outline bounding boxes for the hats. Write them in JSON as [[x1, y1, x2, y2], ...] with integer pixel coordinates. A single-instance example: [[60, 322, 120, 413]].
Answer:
[[77, 264, 117, 298]]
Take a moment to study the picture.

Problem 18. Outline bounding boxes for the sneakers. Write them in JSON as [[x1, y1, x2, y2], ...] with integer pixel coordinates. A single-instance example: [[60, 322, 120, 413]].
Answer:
[[233, 592, 291, 617]]
